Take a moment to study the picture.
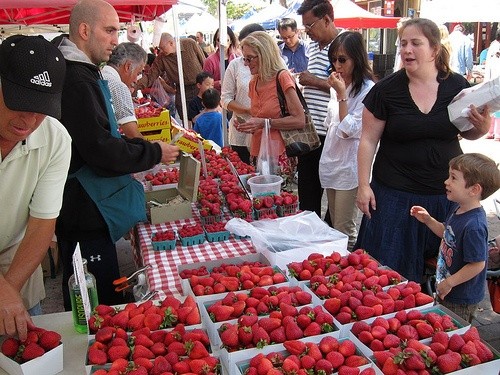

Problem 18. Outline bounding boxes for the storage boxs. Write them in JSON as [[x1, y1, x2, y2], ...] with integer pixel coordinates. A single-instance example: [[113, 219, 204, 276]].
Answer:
[[374, 53, 395, 79], [250, 211, 350, 271], [83, 250, 500, 375], [134, 103, 222, 156], [144, 152, 201, 225], [0, 328, 64, 375]]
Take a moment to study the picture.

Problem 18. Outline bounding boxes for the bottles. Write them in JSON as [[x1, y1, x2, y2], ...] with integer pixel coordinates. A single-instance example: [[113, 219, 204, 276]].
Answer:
[[137, 90, 143, 99], [68, 259, 98, 334]]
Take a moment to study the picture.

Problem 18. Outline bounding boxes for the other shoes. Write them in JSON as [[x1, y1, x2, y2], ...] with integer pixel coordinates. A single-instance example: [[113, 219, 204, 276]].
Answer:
[[487, 136, 494, 139]]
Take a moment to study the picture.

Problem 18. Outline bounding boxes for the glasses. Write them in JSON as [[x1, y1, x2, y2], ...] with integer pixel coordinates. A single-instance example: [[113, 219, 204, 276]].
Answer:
[[243, 55, 259, 63], [329, 56, 353, 63], [305, 14, 327, 30], [282, 31, 296, 41], [215, 38, 231, 42]]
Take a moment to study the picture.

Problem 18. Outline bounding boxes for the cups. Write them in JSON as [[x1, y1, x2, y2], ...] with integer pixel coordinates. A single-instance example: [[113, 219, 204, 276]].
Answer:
[[247, 175, 283, 198]]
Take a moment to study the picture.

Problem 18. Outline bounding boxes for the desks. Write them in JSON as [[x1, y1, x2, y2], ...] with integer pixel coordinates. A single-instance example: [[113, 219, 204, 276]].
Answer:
[[131, 162, 351, 299]]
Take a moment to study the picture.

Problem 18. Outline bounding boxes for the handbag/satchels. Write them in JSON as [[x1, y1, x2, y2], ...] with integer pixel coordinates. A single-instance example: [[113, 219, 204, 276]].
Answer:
[[275, 69, 321, 157], [149, 77, 171, 109], [254, 118, 278, 171]]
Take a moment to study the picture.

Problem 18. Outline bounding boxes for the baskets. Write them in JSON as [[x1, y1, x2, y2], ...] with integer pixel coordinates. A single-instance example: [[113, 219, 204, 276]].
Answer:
[[150, 148, 300, 250]]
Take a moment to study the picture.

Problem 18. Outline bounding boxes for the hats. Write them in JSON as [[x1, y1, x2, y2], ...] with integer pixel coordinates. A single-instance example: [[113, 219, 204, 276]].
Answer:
[[0, 36, 66, 119]]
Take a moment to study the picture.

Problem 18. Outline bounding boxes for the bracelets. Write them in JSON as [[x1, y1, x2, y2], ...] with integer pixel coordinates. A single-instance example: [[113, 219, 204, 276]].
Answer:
[[269, 118, 272, 127], [335, 97, 347, 103]]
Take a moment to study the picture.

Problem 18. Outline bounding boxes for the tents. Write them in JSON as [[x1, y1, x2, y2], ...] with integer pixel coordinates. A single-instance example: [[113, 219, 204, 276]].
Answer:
[[238, 0, 400, 30], [0, 0, 189, 130]]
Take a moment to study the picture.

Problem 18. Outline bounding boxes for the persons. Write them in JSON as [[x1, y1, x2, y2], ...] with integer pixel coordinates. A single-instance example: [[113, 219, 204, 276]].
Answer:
[[318, 32, 376, 253], [447, 24, 473, 81], [220, 23, 266, 170], [100, 43, 148, 140], [128, 18, 309, 150], [234, 31, 306, 191], [409, 153, 500, 325], [479, 28, 500, 140], [0, 36, 72, 343], [51, 0, 181, 311], [296, 0, 345, 229], [352, 19, 490, 295]]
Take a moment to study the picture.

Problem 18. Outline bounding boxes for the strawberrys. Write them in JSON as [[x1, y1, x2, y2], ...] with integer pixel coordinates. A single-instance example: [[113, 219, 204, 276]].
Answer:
[[152, 214, 278, 243], [218, 302, 334, 354], [0, 325, 62, 365], [288, 249, 377, 281], [134, 98, 162, 118], [89, 295, 201, 333], [243, 335, 376, 375], [206, 285, 311, 322], [323, 281, 433, 324], [309, 261, 403, 298], [192, 147, 297, 222], [180, 261, 286, 295], [373, 326, 494, 375], [350, 309, 457, 351], [145, 168, 180, 186], [88, 323, 222, 375]]
